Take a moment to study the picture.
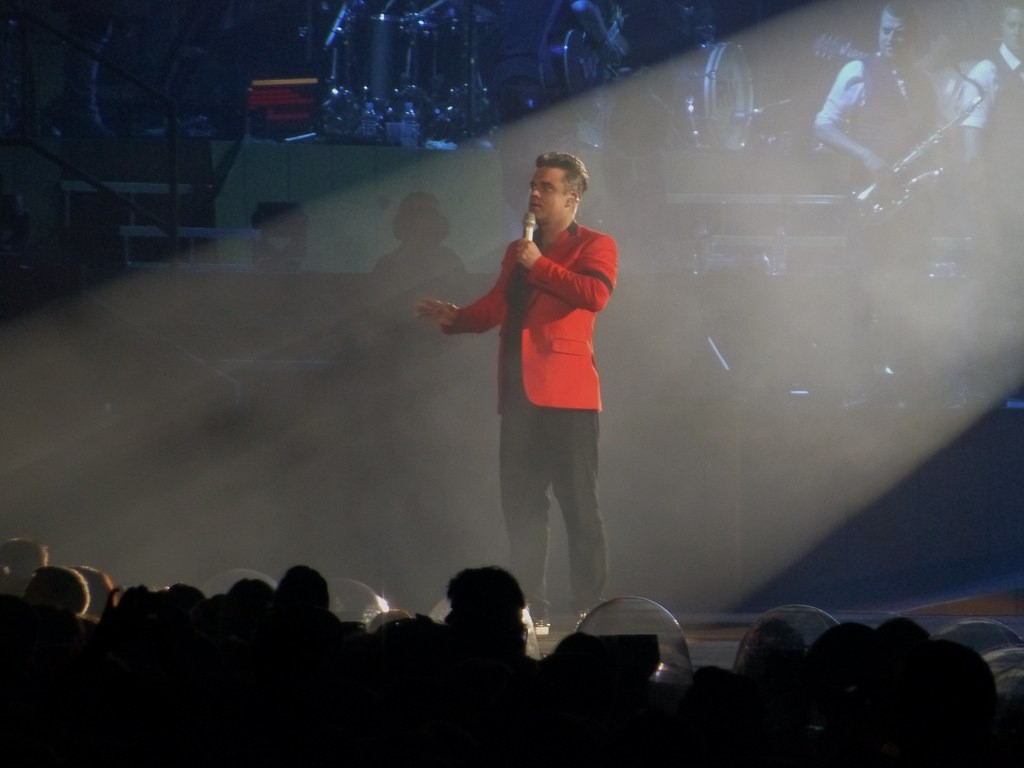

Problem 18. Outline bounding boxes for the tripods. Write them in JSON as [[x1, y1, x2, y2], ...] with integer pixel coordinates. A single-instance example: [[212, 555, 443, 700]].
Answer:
[[317, 0, 495, 148]]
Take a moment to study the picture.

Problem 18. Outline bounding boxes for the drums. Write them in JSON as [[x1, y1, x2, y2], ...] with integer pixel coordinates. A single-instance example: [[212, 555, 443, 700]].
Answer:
[[660, 40, 758, 154]]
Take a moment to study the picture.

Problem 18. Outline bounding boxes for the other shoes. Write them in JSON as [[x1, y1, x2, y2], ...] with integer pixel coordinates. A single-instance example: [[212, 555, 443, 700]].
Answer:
[[524, 601, 550, 635], [575, 607, 591, 632]]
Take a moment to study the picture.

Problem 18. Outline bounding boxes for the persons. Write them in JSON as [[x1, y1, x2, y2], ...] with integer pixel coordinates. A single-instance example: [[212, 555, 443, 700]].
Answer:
[[413, 152, 617, 632], [373, 191, 466, 279], [0, 536, 998, 768], [812, 0, 939, 194], [960, 0, 1024, 164]]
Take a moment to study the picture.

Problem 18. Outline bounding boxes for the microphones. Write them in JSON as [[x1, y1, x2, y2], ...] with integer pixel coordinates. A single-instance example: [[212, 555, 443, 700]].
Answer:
[[518, 211, 537, 279]]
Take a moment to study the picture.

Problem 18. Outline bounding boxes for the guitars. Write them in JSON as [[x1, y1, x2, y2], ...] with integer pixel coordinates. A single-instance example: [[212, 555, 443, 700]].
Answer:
[[545, 6, 630, 130]]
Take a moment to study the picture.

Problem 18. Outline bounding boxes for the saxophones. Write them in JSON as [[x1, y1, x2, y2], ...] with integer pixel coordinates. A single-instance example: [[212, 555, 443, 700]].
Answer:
[[851, 34, 992, 230]]
